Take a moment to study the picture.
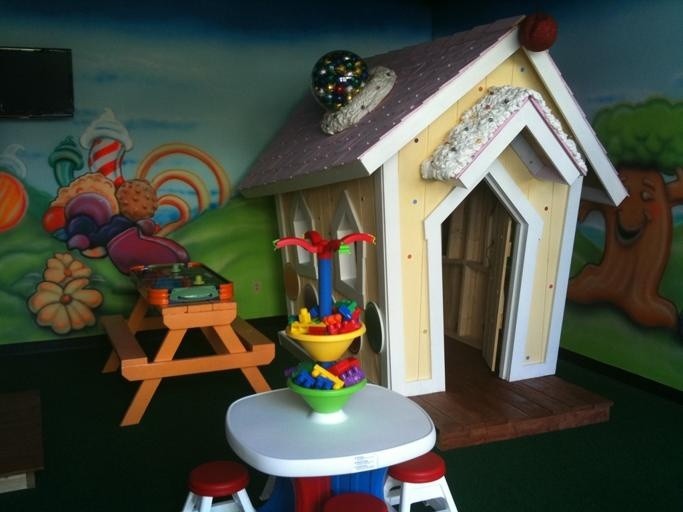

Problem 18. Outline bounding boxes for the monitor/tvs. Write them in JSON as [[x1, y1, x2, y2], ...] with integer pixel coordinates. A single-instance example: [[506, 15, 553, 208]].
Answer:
[[0, 46, 74, 120]]
